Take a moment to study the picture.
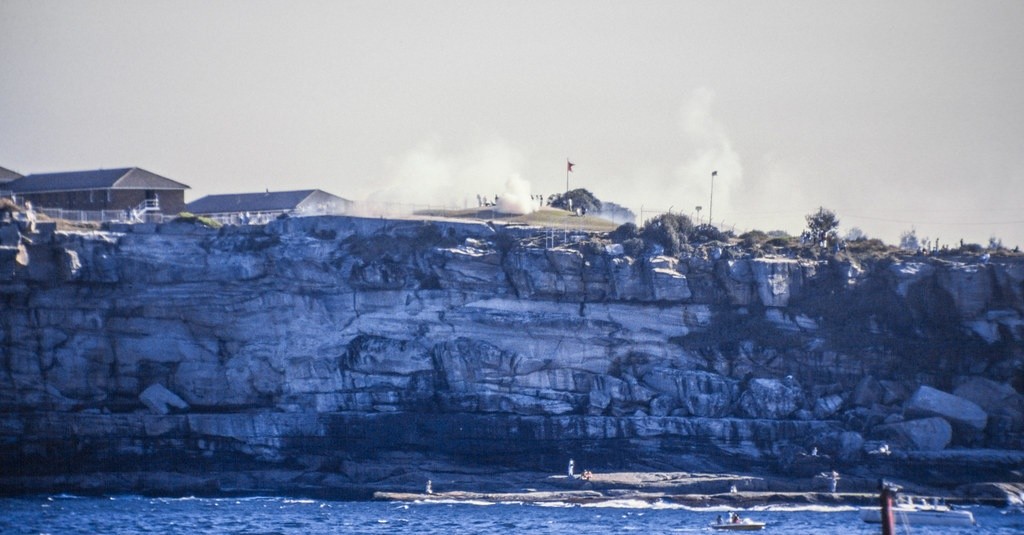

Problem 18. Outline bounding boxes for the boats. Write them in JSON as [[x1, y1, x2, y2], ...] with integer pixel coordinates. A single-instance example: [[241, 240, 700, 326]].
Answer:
[[709, 518, 766, 530], [857, 494, 975, 526]]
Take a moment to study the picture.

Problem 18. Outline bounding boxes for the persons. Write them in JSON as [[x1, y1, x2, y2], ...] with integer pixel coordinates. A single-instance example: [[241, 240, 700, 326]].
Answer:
[[812, 447, 818, 456], [831, 470, 837, 493], [717, 511, 739, 525], [581, 469, 592, 480], [568, 458, 575, 476], [126, 205, 131, 223], [568, 197, 572, 211], [916, 246, 938, 257], [801, 230, 846, 254], [426, 480, 432, 501], [238, 211, 262, 225], [18, 201, 35, 221]]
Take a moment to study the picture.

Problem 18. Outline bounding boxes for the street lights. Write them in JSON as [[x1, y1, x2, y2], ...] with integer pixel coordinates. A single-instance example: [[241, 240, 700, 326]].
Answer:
[[709, 170, 718, 224]]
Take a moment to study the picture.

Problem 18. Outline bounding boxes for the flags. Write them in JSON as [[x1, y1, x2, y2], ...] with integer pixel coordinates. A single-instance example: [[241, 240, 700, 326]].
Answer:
[[568, 162, 575, 172]]
